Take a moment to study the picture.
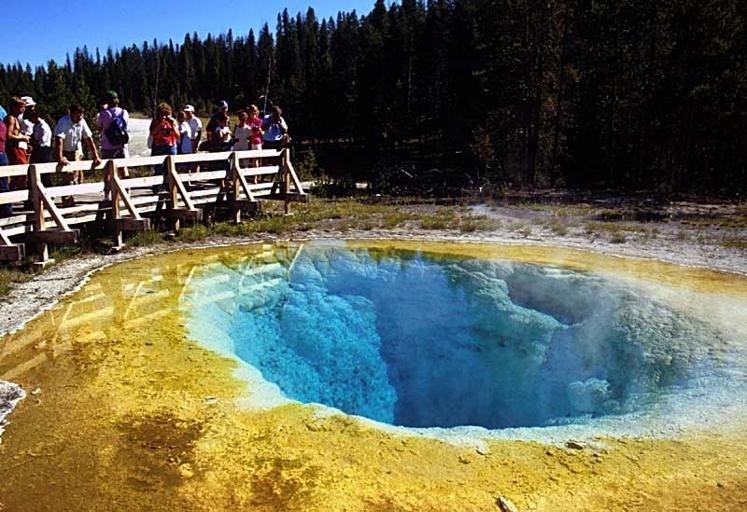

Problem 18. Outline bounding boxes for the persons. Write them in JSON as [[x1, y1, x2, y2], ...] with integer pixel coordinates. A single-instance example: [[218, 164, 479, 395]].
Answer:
[[0, 87, 290, 218]]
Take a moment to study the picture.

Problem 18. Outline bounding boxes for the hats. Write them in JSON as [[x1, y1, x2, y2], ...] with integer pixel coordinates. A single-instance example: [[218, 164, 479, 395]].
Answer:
[[184, 104, 194, 112], [22, 96, 37, 107], [106, 91, 117, 98], [217, 100, 228, 109]]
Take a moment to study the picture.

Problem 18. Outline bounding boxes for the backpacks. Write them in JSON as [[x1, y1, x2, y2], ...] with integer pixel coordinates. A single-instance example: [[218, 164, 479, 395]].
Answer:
[[105, 108, 128, 145]]
